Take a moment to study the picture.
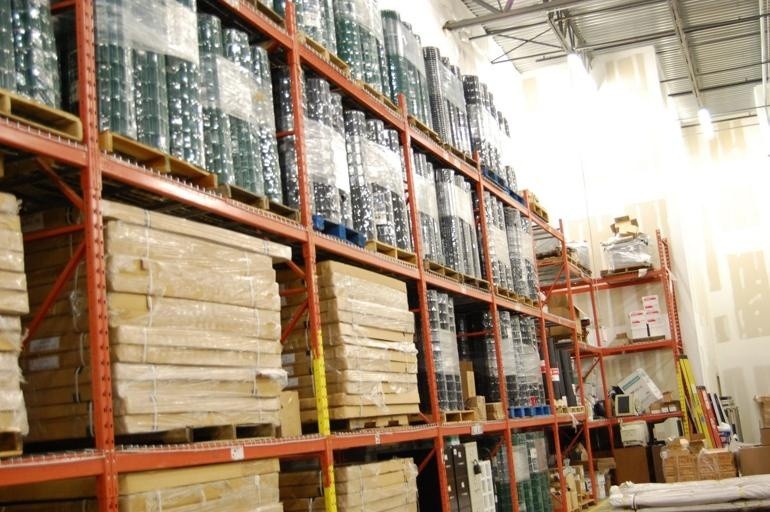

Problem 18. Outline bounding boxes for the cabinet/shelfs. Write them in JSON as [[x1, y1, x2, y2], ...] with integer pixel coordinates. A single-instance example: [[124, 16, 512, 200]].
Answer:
[[0, 1, 598, 512], [541, 231, 691, 483]]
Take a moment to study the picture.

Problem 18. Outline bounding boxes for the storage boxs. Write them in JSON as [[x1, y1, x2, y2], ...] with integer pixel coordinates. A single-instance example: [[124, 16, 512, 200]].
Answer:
[[738, 444, 770, 475], [615, 447, 651, 484], [651, 443, 667, 483]]
